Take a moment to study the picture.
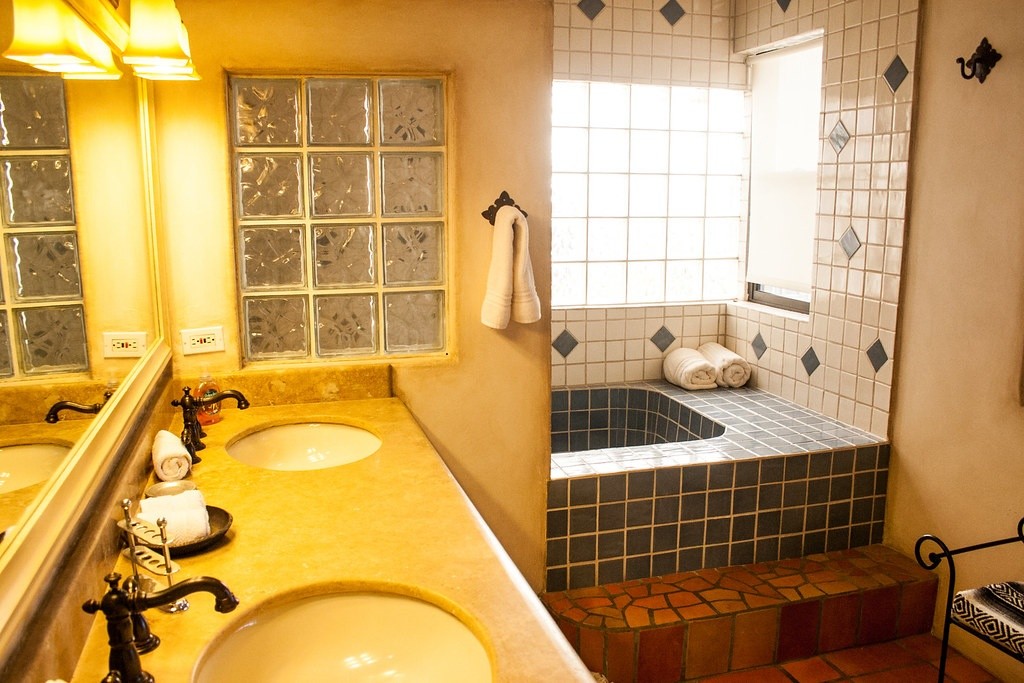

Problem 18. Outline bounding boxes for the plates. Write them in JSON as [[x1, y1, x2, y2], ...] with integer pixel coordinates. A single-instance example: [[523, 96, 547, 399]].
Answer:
[[135, 505, 234, 547], [146, 479, 196, 497]]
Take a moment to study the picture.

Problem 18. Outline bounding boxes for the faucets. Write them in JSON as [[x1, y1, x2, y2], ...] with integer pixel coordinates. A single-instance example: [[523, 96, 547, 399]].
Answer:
[[45, 389, 114, 423], [99, 576, 237, 683], [170, 386, 250, 450]]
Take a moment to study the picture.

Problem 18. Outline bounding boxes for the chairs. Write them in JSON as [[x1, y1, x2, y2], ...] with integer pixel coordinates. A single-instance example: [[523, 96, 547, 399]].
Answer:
[[914, 516, 1024, 683]]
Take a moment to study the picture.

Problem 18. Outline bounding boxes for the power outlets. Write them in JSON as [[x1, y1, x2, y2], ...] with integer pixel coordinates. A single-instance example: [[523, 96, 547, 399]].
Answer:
[[103, 332, 148, 358], [180, 326, 226, 356]]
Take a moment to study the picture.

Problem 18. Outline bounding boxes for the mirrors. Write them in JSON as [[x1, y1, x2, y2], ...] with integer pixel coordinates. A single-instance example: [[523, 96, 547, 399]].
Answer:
[[0, 1, 174, 671]]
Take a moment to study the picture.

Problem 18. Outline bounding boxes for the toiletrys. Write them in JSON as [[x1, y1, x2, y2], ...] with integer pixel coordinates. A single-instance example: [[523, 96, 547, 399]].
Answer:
[[193, 375, 222, 425]]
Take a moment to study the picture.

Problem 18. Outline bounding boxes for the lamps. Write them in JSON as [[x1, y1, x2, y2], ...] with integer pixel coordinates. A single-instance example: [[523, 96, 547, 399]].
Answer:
[[0, 0, 123, 81], [121, 0, 202, 81]]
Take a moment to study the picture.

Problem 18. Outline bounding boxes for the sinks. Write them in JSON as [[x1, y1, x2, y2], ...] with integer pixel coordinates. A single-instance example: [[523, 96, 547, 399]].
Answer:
[[226, 416, 384, 472], [194, 578, 496, 683], [0, 437, 76, 497]]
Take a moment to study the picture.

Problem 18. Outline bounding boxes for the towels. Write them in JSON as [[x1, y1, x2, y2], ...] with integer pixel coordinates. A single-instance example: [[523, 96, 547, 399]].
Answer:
[[152, 431, 192, 480], [480, 205, 541, 329], [137, 490, 209, 543], [697, 344, 750, 388], [663, 347, 717, 389]]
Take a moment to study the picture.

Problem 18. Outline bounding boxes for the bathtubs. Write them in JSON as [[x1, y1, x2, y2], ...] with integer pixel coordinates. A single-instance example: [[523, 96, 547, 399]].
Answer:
[[547, 377, 888, 591]]
